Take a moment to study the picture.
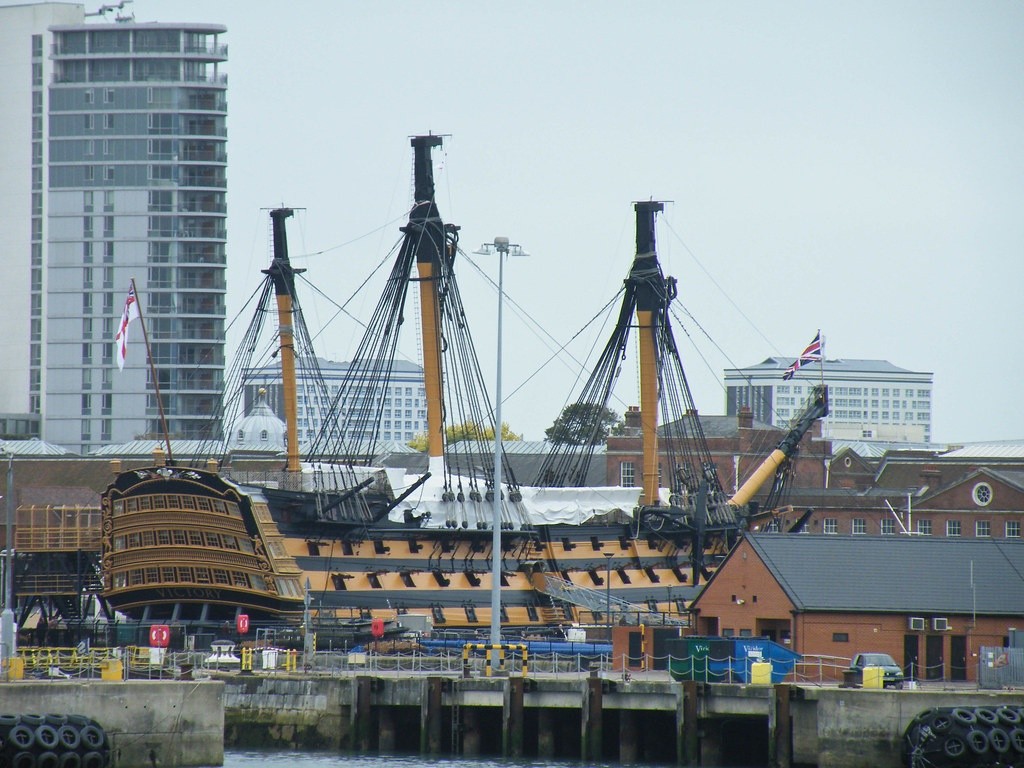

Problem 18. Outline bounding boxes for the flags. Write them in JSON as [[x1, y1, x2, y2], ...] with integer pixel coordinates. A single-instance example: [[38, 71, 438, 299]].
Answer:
[[114, 285, 140, 374], [780, 331, 822, 381]]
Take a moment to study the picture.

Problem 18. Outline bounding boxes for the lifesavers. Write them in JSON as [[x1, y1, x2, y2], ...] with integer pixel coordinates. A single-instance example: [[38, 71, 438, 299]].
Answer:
[[0, 712, 107, 768], [950, 707, 1024, 757]]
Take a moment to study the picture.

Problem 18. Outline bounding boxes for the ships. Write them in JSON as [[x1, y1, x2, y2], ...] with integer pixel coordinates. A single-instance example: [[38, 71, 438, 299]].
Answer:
[[91, 126, 829, 642]]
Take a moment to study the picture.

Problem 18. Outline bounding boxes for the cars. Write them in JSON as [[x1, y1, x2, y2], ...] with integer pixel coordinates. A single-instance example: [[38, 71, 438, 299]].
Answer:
[[853, 653, 904, 689]]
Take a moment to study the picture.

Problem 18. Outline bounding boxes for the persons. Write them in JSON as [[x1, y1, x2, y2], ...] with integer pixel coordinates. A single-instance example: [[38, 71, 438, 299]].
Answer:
[[556, 624, 569, 642]]
